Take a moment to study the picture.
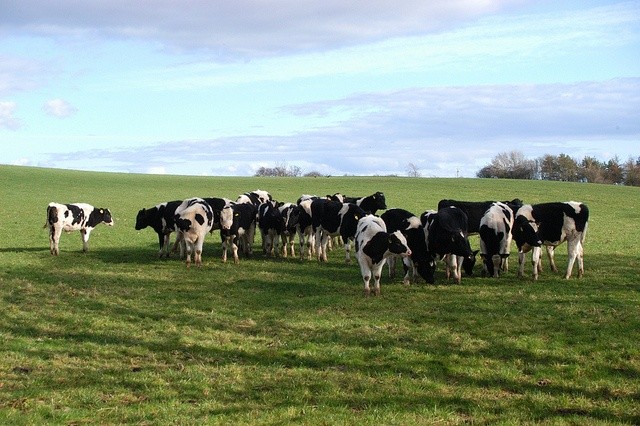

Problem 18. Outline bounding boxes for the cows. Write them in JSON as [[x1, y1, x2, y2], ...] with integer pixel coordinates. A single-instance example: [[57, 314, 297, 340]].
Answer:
[[135, 188, 589, 297], [42, 201, 115, 255]]
